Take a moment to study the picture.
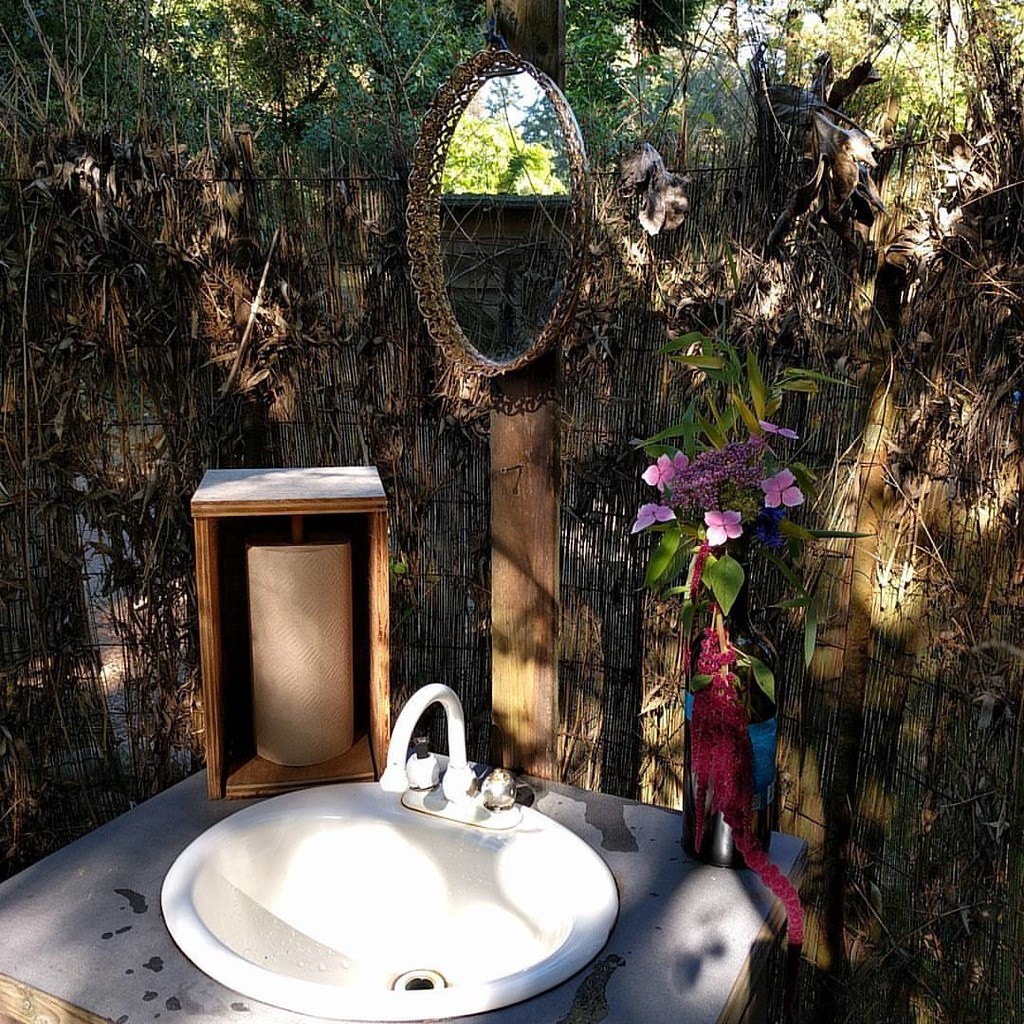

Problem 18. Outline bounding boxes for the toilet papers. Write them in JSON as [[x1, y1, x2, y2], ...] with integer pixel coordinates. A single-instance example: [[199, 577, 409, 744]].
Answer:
[[246, 533, 355, 765]]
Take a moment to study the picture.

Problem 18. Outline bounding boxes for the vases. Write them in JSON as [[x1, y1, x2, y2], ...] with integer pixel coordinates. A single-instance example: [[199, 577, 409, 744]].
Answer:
[[684, 556, 782, 870]]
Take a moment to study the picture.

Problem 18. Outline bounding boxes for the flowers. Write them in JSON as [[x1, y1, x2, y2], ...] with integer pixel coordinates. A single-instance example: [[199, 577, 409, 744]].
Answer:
[[630, 224, 878, 1024]]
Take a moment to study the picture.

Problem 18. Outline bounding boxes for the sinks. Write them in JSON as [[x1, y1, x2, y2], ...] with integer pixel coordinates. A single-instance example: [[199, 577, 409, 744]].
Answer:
[[160, 778, 620, 1024]]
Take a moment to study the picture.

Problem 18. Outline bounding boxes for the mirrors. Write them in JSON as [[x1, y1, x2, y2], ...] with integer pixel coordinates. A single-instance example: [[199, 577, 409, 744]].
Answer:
[[408, 12, 591, 378]]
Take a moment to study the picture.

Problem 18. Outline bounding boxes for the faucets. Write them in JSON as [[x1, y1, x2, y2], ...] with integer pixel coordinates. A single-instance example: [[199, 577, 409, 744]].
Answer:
[[378, 683, 478, 802]]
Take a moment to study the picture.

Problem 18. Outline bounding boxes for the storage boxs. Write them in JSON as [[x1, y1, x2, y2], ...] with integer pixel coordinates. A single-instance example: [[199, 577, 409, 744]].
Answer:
[[192, 466, 390, 800]]
